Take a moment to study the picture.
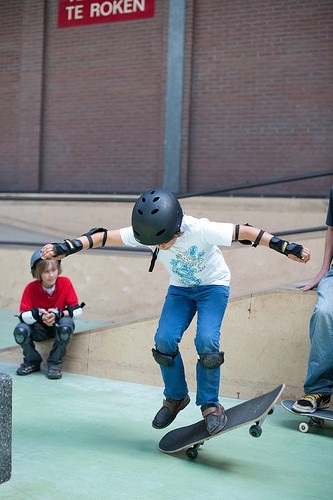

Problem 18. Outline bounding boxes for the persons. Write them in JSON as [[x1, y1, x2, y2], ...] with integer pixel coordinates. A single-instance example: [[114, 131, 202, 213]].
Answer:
[[292, 186, 333, 413], [39, 187, 311, 434], [13, 247, 86, 379]]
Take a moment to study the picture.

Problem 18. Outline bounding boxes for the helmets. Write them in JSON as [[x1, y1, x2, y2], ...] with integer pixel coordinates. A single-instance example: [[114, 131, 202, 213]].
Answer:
[[131, 188, 182, 245], [29, 247, 60, 278]]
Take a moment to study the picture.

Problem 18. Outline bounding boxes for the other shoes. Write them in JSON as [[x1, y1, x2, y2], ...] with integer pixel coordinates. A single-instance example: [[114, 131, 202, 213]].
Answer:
[[47, 368, 61, 379], [200, 403, 227, 435], [151, 395, 190, 429], [15, 363, 39, 375], [292, 392, 331, 413]]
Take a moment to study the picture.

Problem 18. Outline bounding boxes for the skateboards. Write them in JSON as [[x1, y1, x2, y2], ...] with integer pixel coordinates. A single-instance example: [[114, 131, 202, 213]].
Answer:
[[280, 395, 333, 433], [158, 379, 285, 460]]
